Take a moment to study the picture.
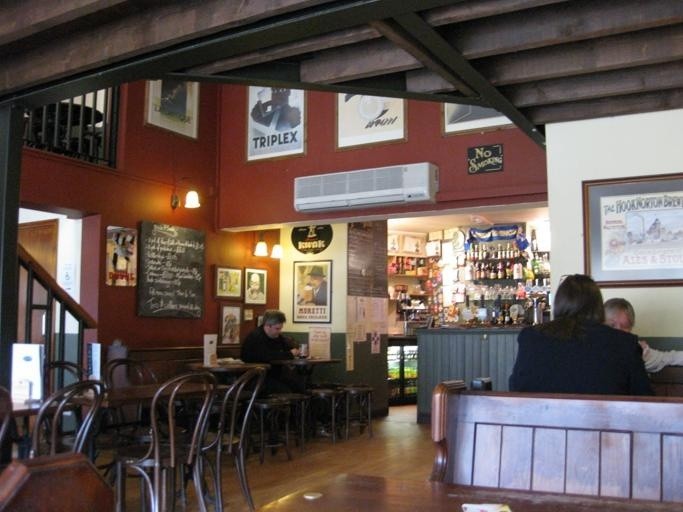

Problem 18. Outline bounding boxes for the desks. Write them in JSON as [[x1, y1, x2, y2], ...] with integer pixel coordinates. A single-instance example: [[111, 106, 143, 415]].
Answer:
[[256, 473, 683, 512], [183, 359, 342, 438]]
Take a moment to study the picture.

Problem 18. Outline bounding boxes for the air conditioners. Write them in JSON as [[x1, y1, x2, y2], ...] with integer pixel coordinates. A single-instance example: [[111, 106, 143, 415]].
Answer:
[[294, 162, 439, 213]]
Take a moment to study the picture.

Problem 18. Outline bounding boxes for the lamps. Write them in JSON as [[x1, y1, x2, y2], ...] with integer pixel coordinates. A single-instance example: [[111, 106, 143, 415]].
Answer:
[[172, 177, 201, 209], [271, 229, 281, 258], [253, 230, 269, 257]]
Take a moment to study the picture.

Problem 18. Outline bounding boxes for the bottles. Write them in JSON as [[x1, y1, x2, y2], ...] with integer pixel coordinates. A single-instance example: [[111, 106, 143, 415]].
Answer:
[[530, 228, 539, 251], [463, 296, 542, 325], [524, 278, 548, 296], [467, 245, 550, 280], [397, 257, 425, 275], [386, 367, 417, 393]]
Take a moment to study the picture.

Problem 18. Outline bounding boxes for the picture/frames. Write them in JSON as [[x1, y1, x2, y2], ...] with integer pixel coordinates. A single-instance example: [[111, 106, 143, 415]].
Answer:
[[292, 259, 332, 324], [218, 301, 242, 345], [334, 92, 409, 153], [440, 102, 518, 138], [243, 267, 268, 306], [213, 264, 244, 302], [143, 78, 201, 143], [244, 84, 307, 165], [582, 172, 683, 289]]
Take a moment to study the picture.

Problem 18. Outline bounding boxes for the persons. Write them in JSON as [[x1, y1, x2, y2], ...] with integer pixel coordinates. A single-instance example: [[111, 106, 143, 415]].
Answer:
[[247, 273, 263, 301], [240, 309, 335, 440], [508, 270, 656, 396], [599, 297, 683, 375], [298, 265, 327, 306]]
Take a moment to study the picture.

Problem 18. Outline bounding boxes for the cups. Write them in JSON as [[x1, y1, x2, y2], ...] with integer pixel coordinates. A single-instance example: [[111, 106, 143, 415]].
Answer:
[[298, 343, 307, 359], [474, 283, 514, 299]]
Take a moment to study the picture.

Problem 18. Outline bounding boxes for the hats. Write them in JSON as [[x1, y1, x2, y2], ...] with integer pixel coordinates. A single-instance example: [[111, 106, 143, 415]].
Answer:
[[308, 266, 325, 276]]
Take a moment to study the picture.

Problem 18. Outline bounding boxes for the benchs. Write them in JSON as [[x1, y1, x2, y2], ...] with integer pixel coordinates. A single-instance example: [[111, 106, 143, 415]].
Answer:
[[429, 379, 683, 502], [129, 345, 242, 424]]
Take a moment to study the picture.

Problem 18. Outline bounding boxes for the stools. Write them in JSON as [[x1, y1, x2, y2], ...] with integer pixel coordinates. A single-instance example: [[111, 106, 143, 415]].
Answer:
[[248, 386, 374, 464]]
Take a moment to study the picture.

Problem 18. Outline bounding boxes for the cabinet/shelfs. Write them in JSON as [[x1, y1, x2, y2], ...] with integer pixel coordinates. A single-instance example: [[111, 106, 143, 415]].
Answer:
[[388, 252, 550, 334], [387, 334, 418, 406]]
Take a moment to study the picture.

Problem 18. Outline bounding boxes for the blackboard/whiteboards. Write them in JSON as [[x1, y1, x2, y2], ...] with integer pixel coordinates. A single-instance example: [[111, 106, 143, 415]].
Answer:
[[136, 220, 205, 319], [347, 219, 390, 298]]
